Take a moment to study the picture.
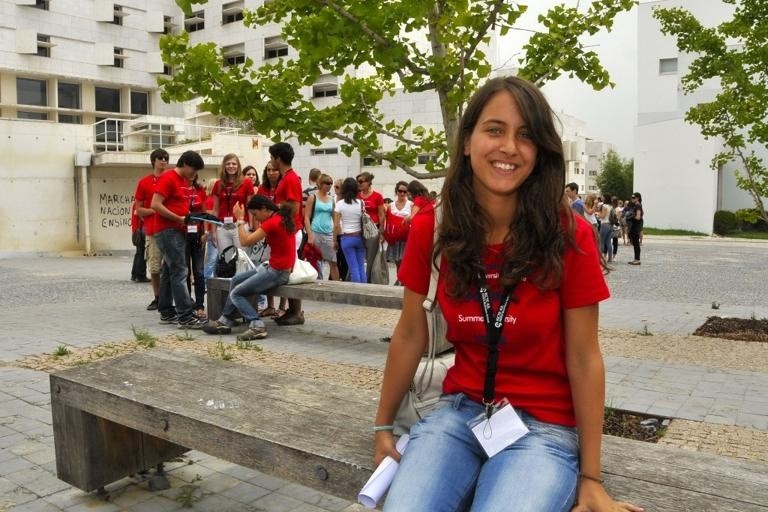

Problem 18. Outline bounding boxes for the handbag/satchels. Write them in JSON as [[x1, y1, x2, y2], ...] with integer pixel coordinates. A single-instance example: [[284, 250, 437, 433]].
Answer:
[[611, 229, 622, 238], [391, 194, 448, 438], [361, 201, 379, 240], [132, 228, 142, 246], [297, 231, 308, 260], [370, 239, 389, 285], [289, 258, 319, 284], [216, 245, 238, 278]]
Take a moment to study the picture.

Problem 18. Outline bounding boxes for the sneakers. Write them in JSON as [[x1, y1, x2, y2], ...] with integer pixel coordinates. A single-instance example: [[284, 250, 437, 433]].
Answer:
[[131, 274, 136, 280], [136, 277, 152, 282], [237, 327, 268, 341], [147, 300, 158, 310], [201, 320, 231, 334], [194, 309, 206, 318], [275, 308, 294, 321], [159, 313, 179, 324], [277, 310, 304, 325], [177, 314, 208, 329]]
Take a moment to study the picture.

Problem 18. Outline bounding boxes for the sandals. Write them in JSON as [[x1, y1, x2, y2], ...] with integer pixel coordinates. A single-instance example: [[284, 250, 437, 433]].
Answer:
[[271, 307, 286, 319], [258, 307, 275, 317]]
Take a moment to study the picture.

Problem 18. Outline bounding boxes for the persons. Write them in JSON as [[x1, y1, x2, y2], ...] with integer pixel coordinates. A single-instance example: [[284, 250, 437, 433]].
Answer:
[[129, 142, 306, 339], [562, 181, 645, 266], [372, 78, 647, 511], [302, 168, 437, 286]]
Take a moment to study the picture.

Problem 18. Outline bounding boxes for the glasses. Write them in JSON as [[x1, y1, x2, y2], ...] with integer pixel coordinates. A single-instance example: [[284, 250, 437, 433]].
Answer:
[[359, 180, 366, 184], [157, 156, 168, 161], [632, 197, 635, 199], [334, 186, 339, 189], [397, 189, 407, 193], [320, 182, 332, 186]]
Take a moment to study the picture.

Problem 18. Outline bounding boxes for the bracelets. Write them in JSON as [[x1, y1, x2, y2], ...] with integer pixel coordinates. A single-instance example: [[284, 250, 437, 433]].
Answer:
[[372, 424, 394, 430], [577, 471, 605, 485]]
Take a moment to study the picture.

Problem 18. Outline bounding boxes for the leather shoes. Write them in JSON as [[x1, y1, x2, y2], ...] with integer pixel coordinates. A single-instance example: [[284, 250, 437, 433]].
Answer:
[[628, 261, 634, 263], [630, 262, 640, 266]]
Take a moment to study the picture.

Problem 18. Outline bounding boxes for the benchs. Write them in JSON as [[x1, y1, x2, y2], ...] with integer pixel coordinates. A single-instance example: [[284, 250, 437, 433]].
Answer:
[[48, 346, 766, 512], [205, 276, 454, 355]]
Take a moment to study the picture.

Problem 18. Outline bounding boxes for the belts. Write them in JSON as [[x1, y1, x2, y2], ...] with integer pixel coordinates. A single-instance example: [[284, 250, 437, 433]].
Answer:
[[339, 233, 361, 237]]
